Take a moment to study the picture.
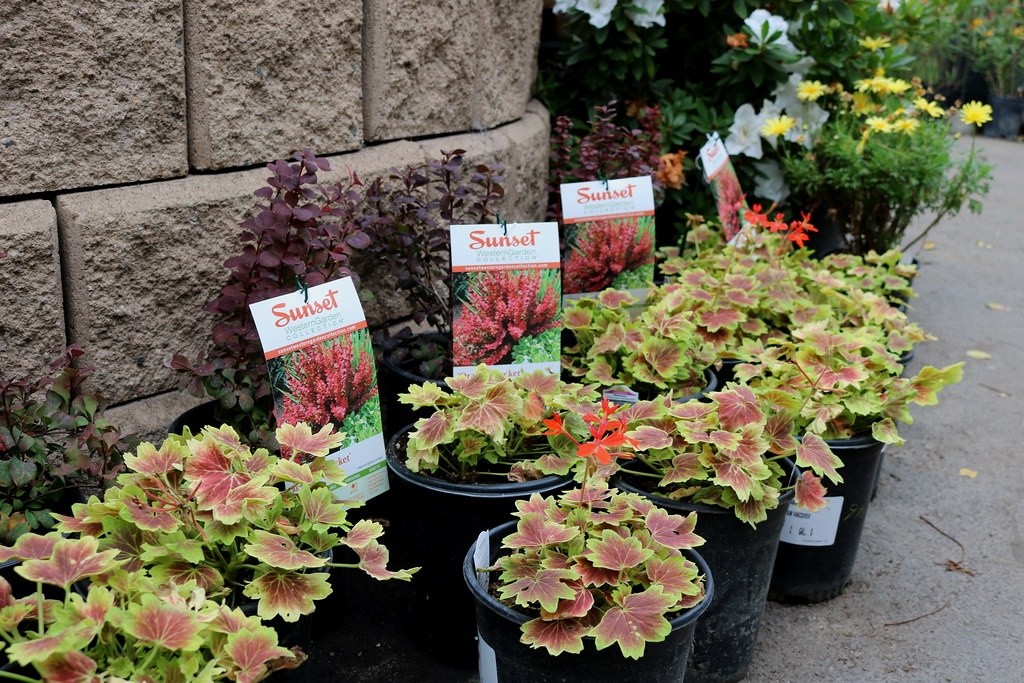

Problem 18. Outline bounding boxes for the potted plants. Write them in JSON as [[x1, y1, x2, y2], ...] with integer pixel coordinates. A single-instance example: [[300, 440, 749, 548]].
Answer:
[[1, 0, 1023, 683]]
